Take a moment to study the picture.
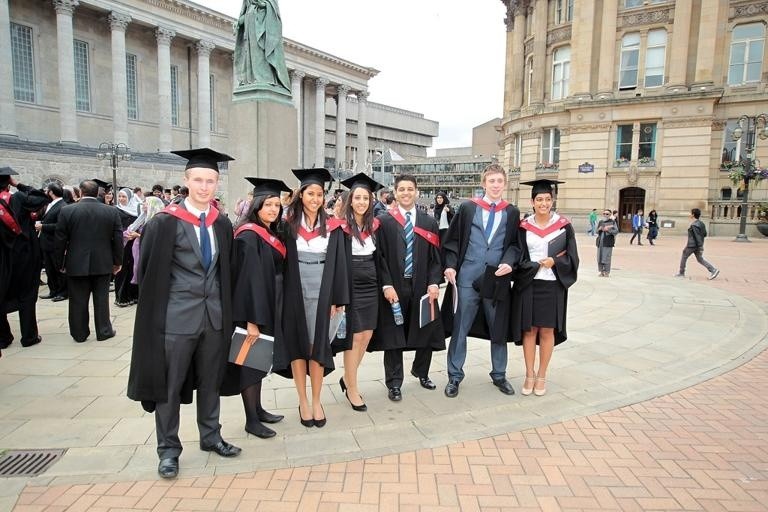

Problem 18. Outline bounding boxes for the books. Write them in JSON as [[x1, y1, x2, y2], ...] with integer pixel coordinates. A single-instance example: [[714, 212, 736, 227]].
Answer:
[[419, 294, 439, 328], [547, 228, 566, 257], [328, 311, 343, 344], [228, 326, 274, 375]]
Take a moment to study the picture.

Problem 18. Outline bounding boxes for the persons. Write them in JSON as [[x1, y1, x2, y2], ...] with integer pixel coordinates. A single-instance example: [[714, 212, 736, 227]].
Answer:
[[646, 210, 659, 244], [413, 203, 434, 217], [115, 188, 142, 306], [442, 164, 519, 397], [35, 183, 68, 301], [92, 178, 115, 206], [373, 191, 390, 216], [231, 177, 286, 437], [134, 187, 142, 199], [150, 184, 188, 206], [586, 209, 597, 236], [434, 192, 454, 243], [596, 209, 618, 278], [127, 148, 241, 477], [331, 172, 382, 411], [515, 178, 578, 396], [123, 196, 165, 285], [235, 191, 253, 228], [629, 209, 644, 245], [54, 180, 124, 342], [285, 168, 349, 426], [1, 166, 48, 349], [367, 175, 446, 401], [673, 208, 720, 280], [327, 189, 344, 210], [387, 196, 396, 213], [62, 184, 80, 203], [611, 211, 619, 227]]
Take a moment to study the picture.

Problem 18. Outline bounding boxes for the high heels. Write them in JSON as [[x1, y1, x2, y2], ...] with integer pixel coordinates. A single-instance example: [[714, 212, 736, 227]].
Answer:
[[339, 376, 363, 399], [346, 388, 367, 411]]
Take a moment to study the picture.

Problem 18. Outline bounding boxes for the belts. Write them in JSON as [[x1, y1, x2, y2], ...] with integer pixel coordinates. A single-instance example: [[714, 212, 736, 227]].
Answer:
[[298, 260, 326, 264], [351, 258, 373, 262]]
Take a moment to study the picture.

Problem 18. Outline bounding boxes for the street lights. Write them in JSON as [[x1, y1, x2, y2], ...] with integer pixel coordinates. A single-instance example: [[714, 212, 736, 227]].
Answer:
[[731, 114, 768, 242], [96, 143, 132, 207], [330, 161, 353, 191]]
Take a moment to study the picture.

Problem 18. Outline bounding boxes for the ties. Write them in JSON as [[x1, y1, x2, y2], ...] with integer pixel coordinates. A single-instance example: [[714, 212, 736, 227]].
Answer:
[[403, 212, 415, 275], [200, 213, 211, 270], [484, 203, 496, 241]]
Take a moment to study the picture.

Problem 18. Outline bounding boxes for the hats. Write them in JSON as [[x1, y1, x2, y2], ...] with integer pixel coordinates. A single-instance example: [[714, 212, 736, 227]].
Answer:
[[93, 179, 108, 187], [1, 166, 19, 188], [519, 179, 565, 193], [245, 177, 292, 197], [165, 189, 171, 193], [340, 172, 385, 192], [291, 168, 335, 187], [169, 148, 236, 174], [472, 265, 512, 306]]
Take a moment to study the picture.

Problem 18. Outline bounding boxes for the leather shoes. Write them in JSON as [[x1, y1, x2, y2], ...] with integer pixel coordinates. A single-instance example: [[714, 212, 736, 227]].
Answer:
[[39, 294, 53, 298], [259, 414, 284, 423], [314, 403, 326, 428], [533, 378, 546, 396], [493, 378, 514, 395], [245, 423, 276, 438], [111, 330, 116, 337], [299, 405, 315, 427], [22, 335, 41, 347], [521, 377, 535, 396], [444, 379, 460, 398], [52, 296, 69, 301], [201, 439, 242, 457], [388, 386, 401, 401], [411, 368, 436, 389], [158, 455, 178, 478]]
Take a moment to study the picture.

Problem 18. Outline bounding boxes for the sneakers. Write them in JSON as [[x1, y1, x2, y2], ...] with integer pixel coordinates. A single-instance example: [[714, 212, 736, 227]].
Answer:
[[708, 269, 718, 280]]
